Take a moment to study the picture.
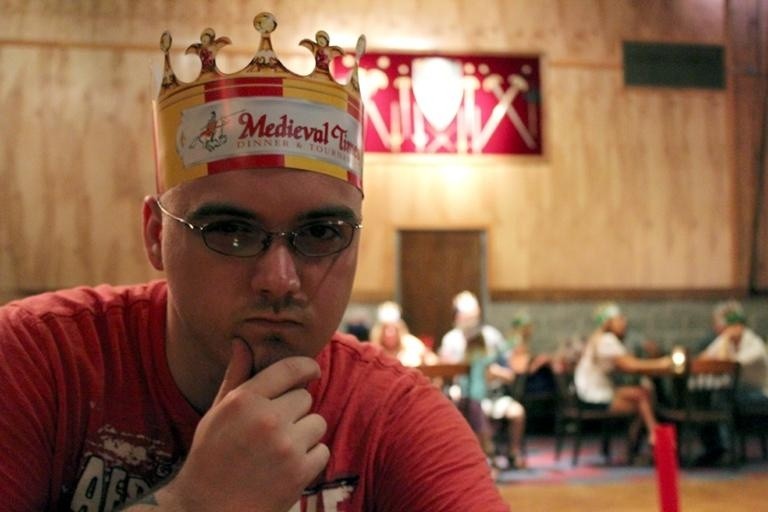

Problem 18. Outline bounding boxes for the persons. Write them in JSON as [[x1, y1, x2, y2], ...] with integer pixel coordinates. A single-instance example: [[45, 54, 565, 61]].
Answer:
[[0, 12, 509, 512], [505, 309, 542, 372], [347, 308, 368, 341], [576, 300, 658, 466], [687, 297, 768, 472], [368, 309, 437, 368], [432, 290, 526, 470]]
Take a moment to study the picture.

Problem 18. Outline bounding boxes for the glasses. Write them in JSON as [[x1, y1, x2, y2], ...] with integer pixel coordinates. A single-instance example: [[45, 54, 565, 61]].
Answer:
[[155, 197, 364, 261]]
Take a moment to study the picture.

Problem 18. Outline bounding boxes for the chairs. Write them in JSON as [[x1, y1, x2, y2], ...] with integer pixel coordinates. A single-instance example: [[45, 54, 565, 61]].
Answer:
[[414, 358, 742, 472]]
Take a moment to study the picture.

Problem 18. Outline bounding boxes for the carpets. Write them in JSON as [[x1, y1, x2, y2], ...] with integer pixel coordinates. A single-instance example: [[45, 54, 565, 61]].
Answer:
[[482, 433, 768, 487]]
